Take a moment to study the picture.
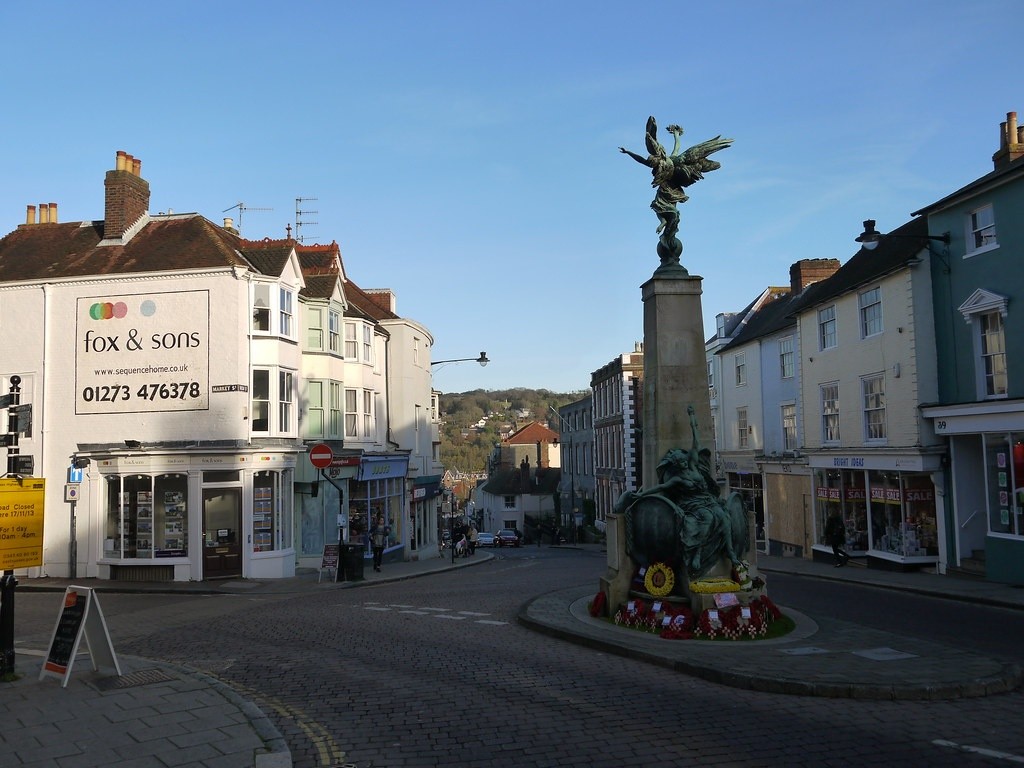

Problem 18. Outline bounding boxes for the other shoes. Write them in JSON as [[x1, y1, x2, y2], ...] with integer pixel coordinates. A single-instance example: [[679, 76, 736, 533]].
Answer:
[[374, 565, 381, 571]]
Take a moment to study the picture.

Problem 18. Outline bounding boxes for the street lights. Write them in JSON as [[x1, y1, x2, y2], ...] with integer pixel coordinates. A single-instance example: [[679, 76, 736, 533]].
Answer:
[[552, 437, 576, 544]]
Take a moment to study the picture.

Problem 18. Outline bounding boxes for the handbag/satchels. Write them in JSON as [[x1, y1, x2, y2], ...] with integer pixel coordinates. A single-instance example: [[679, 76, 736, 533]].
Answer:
[[466, 535, 471, 540]]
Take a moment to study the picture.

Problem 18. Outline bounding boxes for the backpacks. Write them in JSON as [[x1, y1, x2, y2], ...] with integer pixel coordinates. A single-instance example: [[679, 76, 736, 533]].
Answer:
[[833, 522, 845, 537]]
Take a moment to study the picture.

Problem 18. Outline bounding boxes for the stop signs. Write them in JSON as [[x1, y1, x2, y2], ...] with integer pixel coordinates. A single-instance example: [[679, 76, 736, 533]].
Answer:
[[309, 444, 334, 470]]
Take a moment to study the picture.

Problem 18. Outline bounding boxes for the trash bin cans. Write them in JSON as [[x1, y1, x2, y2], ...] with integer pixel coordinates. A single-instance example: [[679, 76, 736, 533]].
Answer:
[[339, 543, 365, 580]]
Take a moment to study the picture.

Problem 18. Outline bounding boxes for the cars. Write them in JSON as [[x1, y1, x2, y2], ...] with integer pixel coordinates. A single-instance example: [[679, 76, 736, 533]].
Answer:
[[476, 533, 495, 548], [493, 530, 519, 548]]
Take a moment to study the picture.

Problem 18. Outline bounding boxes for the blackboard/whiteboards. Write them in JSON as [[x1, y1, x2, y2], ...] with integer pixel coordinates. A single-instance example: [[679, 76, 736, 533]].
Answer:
[[43, 586, 91, 679]]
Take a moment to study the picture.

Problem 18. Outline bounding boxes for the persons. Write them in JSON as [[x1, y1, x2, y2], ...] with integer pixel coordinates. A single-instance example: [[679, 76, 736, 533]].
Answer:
[[825, 507, 850, 568], [629, 405, 742, 568], [619, 116, 732, 235], [468, 524, 478, 556], [368, 516, 390, 573]]
